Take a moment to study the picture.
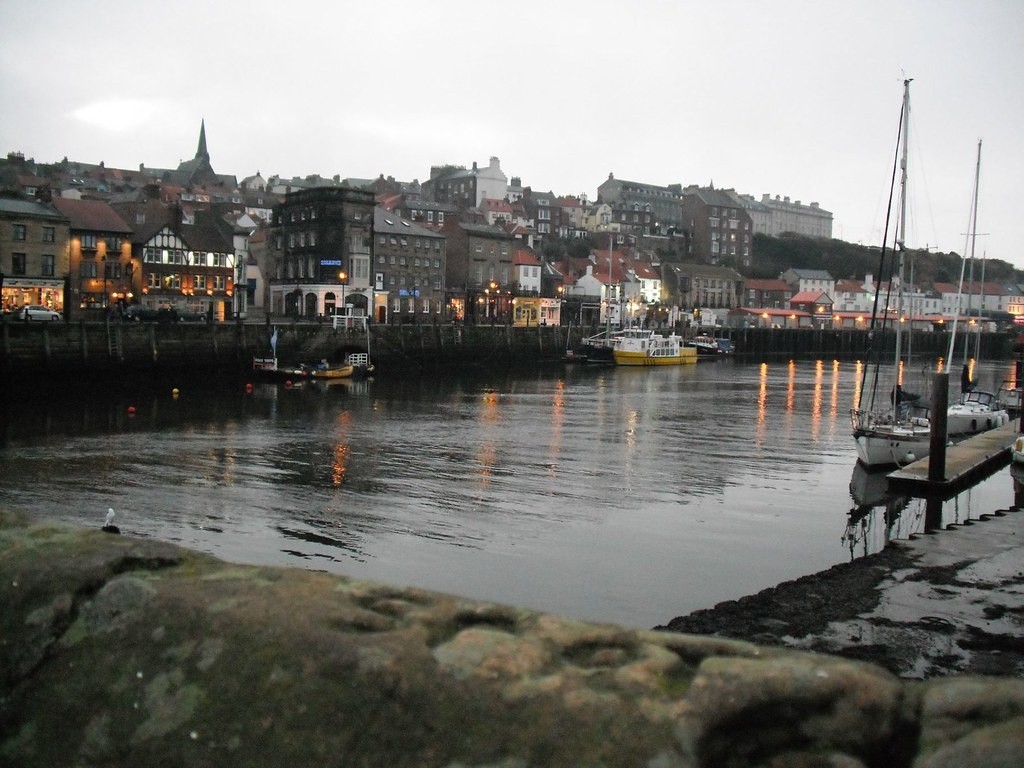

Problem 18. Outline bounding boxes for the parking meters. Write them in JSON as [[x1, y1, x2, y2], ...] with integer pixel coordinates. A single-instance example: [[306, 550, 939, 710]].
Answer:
[[485, 283, 500, 330]]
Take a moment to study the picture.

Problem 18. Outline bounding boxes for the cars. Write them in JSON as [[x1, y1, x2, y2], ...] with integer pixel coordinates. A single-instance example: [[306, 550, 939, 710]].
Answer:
[[15, 305, 61, 322]]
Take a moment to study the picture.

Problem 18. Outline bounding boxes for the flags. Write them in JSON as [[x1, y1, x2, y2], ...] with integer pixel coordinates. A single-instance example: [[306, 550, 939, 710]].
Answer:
[[271, 330, 278, 350]]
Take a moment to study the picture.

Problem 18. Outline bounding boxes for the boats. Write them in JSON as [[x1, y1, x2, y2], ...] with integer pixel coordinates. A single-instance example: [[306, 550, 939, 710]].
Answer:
[[276, 359, 354, 377], [579, 235, 735, 365]]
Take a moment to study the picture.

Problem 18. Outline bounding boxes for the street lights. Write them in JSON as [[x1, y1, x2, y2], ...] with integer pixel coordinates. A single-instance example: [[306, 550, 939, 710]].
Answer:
[[339, 272, 347, 315], [113, 291, 133, 323]]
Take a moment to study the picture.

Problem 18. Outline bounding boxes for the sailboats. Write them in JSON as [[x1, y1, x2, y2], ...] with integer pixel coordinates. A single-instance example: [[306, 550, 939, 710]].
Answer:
[[849, 79, 1024, 475], [842, 457, 971, 561]]
